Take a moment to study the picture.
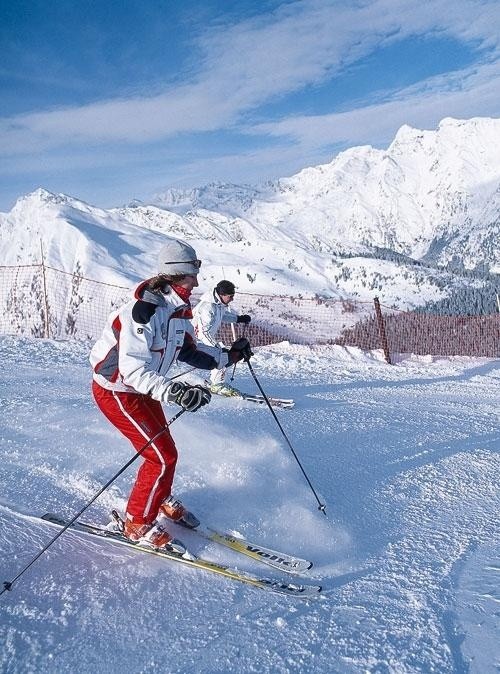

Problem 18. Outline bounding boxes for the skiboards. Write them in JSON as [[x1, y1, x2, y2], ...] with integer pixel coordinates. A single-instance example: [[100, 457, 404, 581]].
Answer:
[[0, 474, 322, 598], [204, 378, 295, 409]]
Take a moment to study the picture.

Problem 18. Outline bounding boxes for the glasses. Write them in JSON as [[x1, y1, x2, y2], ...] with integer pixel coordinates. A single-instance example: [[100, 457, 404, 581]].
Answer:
[[165, 258, 201, 269]]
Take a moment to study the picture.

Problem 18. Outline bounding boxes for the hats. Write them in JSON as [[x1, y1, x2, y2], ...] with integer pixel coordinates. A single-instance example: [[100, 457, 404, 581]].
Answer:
[[159, 239, 200, 277], [216, 280, 235, 296]]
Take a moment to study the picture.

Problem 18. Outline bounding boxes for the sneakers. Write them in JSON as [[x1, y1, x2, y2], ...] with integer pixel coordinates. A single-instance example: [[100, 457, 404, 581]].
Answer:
[[212, 384, 239, 395], [161, 496, 185, 522], [124, 519, 172, 547]]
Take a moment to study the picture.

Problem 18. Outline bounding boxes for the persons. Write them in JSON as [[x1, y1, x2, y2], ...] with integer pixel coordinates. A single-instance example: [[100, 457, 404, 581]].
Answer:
[[87, 239, 254, 562], [188, 280, 251, 397]]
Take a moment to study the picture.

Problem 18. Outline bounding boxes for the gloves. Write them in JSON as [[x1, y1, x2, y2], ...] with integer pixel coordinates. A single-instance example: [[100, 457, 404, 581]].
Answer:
[[225, 337, 254, 370], [236, 313, 251, 325], [166, 380, 212, 414]]
